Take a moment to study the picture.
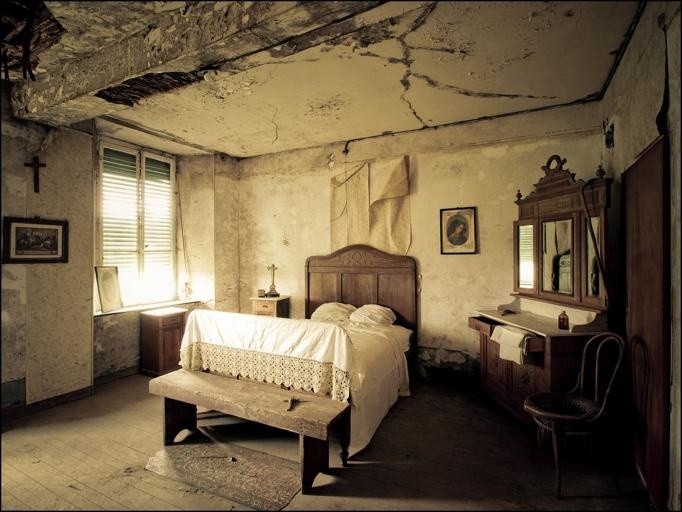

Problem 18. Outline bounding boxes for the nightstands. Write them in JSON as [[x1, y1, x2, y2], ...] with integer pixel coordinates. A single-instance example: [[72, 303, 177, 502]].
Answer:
[[250, 295, 289, 319]]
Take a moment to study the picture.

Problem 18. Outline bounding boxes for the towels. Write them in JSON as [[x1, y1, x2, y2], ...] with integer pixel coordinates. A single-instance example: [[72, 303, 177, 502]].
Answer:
[[489, 326, 536, 366]]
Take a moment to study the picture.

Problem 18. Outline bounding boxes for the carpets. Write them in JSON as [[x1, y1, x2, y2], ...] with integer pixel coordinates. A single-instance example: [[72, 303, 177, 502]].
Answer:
[[146, 425, 303, 512]]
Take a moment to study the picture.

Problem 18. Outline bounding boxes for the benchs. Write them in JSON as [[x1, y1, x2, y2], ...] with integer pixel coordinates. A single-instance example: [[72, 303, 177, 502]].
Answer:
[[149, 369, 351, 495]]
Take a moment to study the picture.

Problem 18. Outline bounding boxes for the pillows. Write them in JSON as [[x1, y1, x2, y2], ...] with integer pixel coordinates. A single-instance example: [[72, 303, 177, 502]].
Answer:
[[311, 303, 357, 321], [348, 303, 397, 326]]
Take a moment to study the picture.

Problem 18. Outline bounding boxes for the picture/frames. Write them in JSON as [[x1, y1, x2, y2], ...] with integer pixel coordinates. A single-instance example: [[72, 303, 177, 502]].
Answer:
[[3, 216, 68, 264], [95, 266, 123, 313], [440, 208, 478, 255]]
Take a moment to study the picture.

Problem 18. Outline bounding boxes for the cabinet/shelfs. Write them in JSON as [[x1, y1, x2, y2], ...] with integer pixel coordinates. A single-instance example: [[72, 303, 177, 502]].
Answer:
[[468, 295, 627, 442], [140, 306, 188, 378]]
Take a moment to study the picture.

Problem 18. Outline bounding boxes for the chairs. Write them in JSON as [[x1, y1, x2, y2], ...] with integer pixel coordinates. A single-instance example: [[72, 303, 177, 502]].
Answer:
[[524, 332, 631, 499]]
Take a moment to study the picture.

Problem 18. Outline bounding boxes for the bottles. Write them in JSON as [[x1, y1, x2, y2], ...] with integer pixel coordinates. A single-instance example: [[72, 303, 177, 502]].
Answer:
[[558, 310, 569, 330]]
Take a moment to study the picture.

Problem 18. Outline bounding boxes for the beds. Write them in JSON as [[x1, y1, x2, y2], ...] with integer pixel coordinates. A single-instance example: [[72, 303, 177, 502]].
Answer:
[[182, 245, 417, 458]]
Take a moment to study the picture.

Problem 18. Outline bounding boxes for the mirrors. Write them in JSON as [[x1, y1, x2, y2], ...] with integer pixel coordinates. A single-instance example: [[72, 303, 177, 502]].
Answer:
[[580, 211, 607, 309], [514, 222, 536, 293], [541, 216, 580, 303]]
